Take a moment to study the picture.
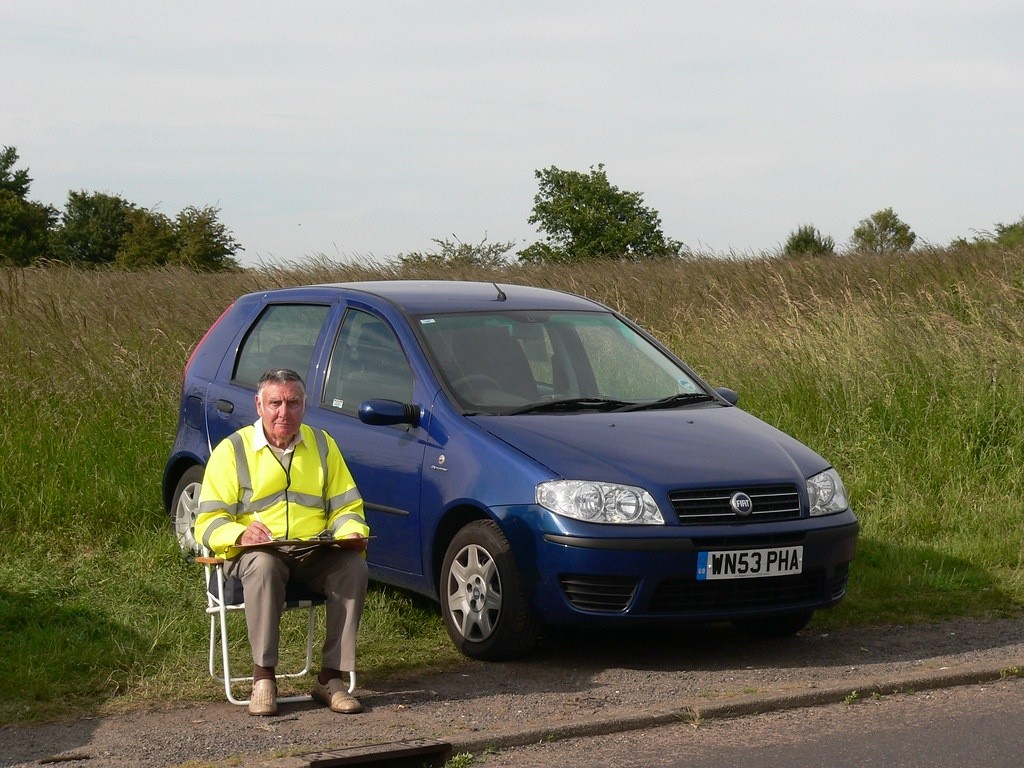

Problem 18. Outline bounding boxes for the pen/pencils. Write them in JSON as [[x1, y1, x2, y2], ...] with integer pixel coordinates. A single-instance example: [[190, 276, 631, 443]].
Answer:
[[253, 510, 273, 542]]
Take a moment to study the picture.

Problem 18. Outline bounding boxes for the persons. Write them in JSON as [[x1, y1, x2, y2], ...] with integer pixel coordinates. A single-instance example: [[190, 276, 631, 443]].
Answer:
[[194, 369, 371, 716]]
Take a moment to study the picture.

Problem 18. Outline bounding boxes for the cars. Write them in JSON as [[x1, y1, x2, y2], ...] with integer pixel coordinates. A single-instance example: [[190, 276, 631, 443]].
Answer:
[[161, 280, 860, 663]]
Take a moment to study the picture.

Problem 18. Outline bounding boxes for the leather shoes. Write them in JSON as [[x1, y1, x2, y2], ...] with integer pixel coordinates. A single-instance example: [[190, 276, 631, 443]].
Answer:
[[248, 677, 278, 716], [311, 678, 364, 714]]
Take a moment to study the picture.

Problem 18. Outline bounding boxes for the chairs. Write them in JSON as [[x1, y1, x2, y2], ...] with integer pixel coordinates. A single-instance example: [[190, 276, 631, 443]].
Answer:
[[194, 545, 356, 704], [460, 326, 538, 396], [351, 321, 414, 401]]
